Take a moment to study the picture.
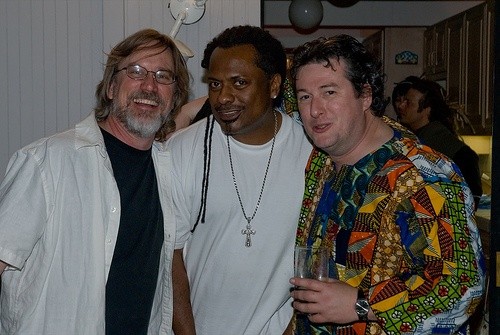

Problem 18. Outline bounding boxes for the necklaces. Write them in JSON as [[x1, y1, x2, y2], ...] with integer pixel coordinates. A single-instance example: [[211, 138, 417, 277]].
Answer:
[[227, 110, 277, 248]]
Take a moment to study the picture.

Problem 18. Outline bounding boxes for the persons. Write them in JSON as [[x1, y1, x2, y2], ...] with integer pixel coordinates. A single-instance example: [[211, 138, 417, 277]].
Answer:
[[0, 25, 485, 335]]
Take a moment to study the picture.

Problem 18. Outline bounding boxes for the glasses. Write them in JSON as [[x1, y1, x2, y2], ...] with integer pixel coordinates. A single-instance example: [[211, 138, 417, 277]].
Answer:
[[111, 64, 178, 85]]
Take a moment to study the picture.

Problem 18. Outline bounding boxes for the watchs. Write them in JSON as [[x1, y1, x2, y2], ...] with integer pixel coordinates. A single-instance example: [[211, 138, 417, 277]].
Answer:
[[355, 290, 369, 322]]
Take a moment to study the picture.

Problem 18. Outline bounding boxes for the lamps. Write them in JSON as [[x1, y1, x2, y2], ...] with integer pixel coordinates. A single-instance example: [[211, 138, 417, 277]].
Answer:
[[167, 0, 208, 57], [289, 0, 323, 29]]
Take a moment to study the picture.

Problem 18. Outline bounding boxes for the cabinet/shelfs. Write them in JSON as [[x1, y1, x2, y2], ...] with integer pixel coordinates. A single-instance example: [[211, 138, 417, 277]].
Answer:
[[362, 2, 491, 274]]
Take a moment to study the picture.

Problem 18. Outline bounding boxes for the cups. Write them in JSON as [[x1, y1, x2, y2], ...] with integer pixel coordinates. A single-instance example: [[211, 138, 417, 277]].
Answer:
[[294, 246, 329, 314]]
